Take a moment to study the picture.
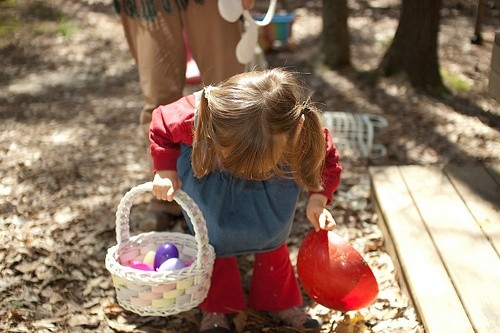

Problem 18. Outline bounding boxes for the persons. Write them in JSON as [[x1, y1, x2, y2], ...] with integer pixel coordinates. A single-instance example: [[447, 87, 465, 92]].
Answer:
[[114, 0, 256, 233], [148, 71, 342, 333]]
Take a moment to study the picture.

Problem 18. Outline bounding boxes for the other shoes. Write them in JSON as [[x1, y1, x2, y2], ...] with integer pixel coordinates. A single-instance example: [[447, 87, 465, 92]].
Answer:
[[273, 308, 322, 332], [199, 313, 249, 333]]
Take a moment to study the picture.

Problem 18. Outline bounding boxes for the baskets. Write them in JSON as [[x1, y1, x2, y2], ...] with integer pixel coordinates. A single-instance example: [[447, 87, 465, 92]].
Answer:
[[104, 181, 216, 317], [324, 109, 388, 160]]
